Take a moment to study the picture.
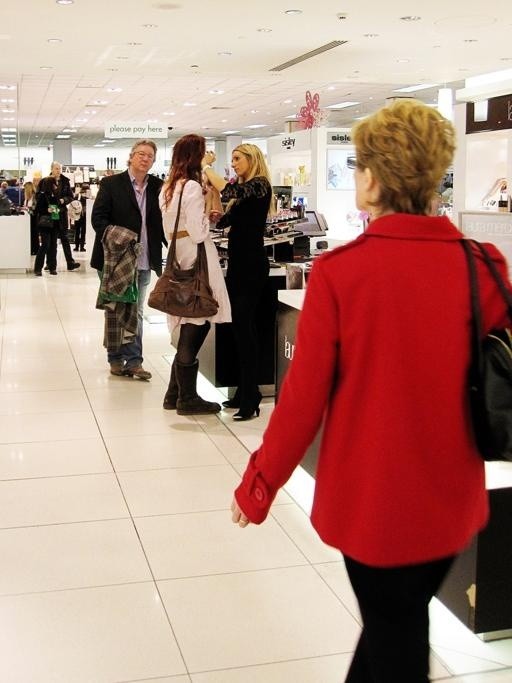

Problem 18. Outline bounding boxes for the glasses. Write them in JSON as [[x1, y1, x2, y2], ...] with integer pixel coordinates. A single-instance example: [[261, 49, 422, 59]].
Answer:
[[347, 155, 367, 172], [137, 151, 154, 160]]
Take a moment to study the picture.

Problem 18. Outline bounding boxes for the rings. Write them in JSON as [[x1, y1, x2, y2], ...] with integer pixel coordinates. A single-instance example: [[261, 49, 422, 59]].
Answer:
[[239, 518, 248, 524]]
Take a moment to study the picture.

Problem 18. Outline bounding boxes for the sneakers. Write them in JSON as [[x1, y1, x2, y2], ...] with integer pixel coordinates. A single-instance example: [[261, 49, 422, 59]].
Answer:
[[111, 364, 152, 379], [31, 247, 86, 276]]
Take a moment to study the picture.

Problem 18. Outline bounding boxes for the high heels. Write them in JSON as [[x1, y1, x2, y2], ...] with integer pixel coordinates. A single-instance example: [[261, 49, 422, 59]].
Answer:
[[222, 386, 262, 421]]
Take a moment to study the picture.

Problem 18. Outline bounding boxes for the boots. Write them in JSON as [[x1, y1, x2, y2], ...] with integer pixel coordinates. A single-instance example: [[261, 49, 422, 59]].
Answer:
[[163, 358, 221, 414]]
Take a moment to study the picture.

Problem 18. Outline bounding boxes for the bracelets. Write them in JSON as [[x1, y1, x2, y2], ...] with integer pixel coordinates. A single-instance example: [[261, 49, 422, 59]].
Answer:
[[202, 163, 215, 170]]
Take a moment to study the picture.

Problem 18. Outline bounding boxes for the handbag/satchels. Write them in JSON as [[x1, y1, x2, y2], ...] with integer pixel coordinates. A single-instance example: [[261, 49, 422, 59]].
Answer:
[[38, 215, 54, 228], [62, 166, 75, 188], [465, 326, 512, 461], [74, 166, 83, 183], [148, 268, 219, 318]]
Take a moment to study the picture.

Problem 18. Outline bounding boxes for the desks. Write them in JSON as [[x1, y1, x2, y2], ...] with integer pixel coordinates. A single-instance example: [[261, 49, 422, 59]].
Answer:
[[167, 270, 286, 388], [0, 209, 33, 275], [429, 462, 509, 645], [273, 289, 328, 483]]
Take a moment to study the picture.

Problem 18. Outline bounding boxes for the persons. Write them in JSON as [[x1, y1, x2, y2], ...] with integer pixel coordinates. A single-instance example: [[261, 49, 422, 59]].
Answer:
[[155, 133, 223, 416], [87, 138, 169, 383], [198, 142, 278, 423], [230, 100, 512, 683], [0, 159, 91, 275]]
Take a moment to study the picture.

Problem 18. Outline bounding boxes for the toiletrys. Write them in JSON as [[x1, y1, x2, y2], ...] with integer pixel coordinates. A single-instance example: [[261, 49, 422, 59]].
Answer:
[[264, 194, 306, 239]]
